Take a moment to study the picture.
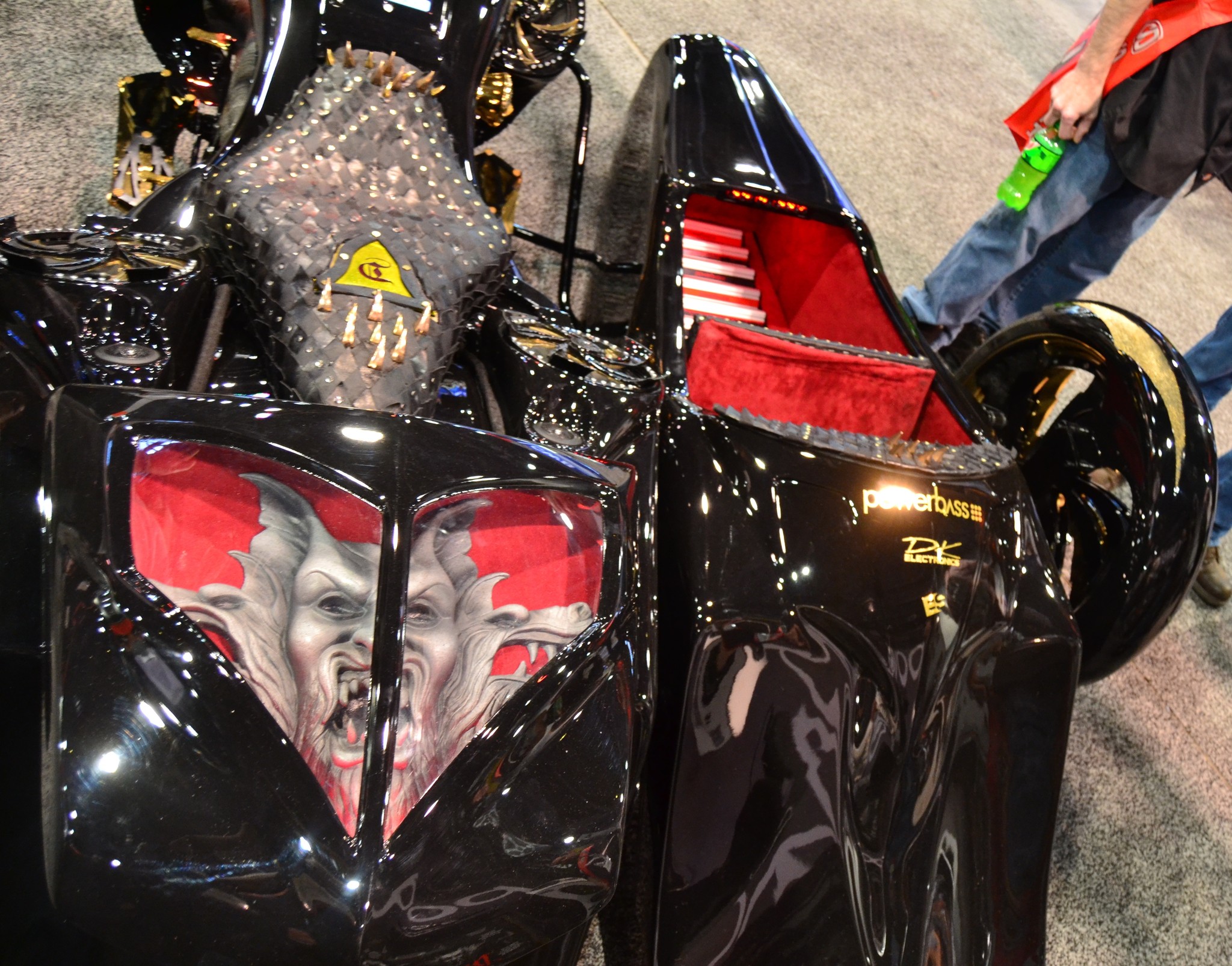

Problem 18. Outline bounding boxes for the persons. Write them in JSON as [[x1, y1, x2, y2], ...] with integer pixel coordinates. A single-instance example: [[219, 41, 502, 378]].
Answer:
[[901, 0, 1232, 372], [1182, 300, 1231, 608]]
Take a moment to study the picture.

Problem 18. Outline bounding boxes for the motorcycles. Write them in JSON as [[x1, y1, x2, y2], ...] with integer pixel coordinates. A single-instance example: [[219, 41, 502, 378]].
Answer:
[[0, 1, 1217, 965]]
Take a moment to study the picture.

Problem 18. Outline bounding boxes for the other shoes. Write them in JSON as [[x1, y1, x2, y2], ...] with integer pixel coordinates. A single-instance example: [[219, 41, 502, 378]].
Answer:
[[953, 322, 987, 365]]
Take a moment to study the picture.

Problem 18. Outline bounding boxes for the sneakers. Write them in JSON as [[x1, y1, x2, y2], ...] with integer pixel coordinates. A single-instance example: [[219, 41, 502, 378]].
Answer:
[[1193, 547, 1231, 608]]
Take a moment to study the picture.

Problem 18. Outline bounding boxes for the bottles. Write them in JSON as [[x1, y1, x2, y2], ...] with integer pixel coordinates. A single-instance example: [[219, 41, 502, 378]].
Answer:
[[996, 115, 1071, 213]]
